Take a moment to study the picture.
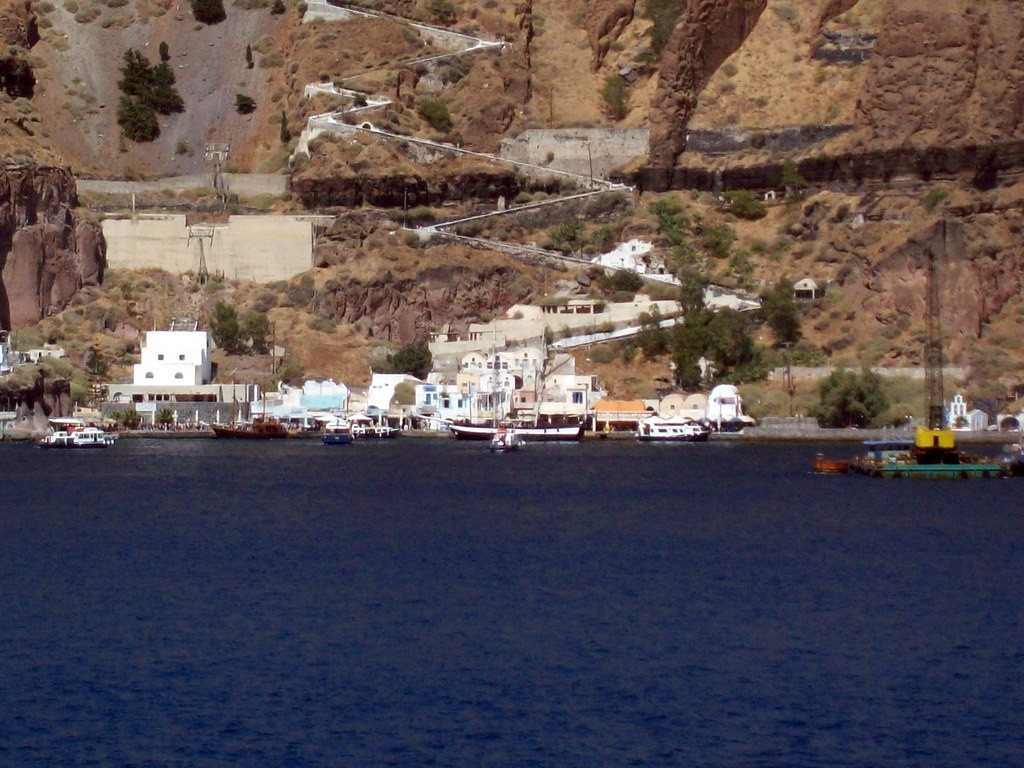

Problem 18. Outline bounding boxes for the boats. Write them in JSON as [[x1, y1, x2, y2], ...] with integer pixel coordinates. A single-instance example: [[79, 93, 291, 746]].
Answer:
[[321, 433, 353, 445], [635, 416, 712, 442], [447, 421, 586, 441], [37, 427, 116, 449], [325, 424, 400, 439], [488, 421, 526, 453], [212, 423, 290, 439]]
